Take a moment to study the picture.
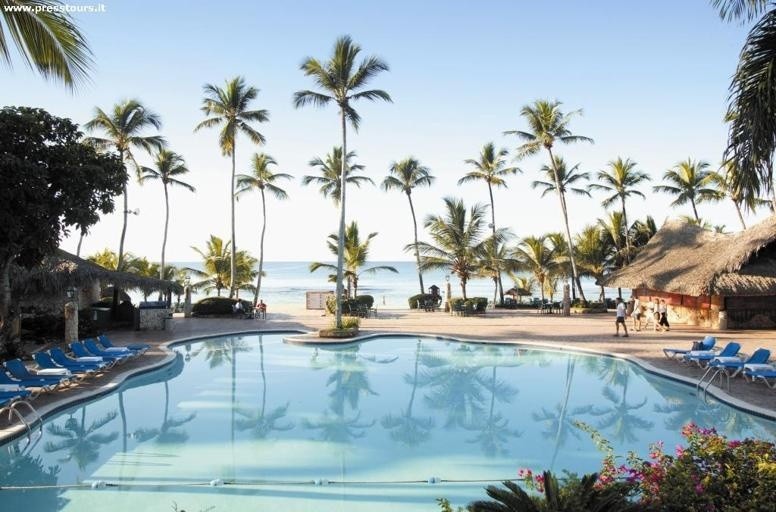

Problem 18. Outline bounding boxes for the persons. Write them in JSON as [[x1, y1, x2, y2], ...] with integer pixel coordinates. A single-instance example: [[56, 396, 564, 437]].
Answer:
[[613, 297, 629, 338], [659, 299, 670, 331], [255, 299, 266, 317], [651, 298, 667, 333], [629, 295, 643, 332], [642, 296, 655, 330], [235, 299, 245, 314]]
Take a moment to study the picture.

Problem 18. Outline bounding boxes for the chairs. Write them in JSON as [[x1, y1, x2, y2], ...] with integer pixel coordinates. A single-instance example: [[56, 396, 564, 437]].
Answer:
[[661, 335, 776, 391], [0, 335, 151, 424]]
